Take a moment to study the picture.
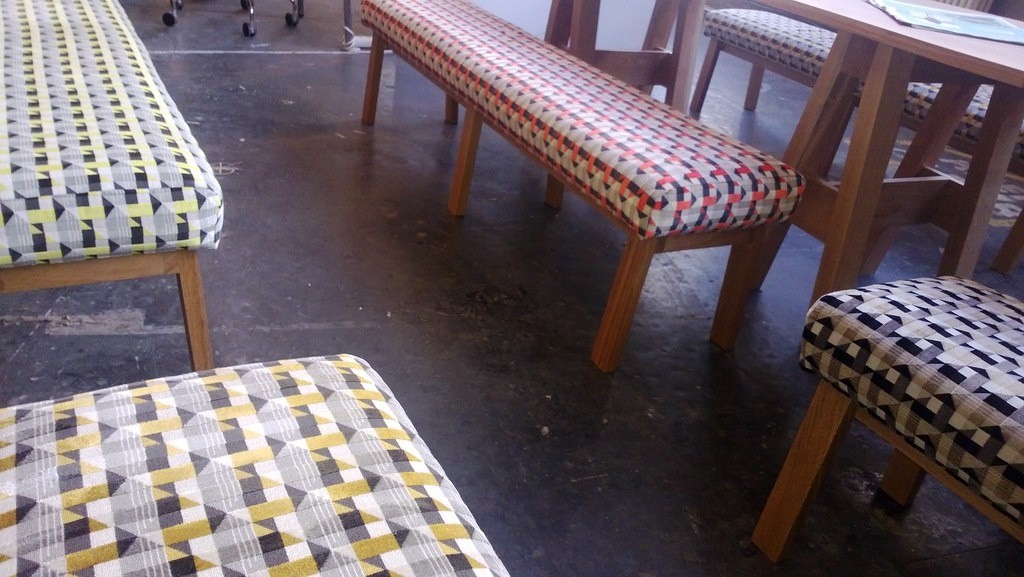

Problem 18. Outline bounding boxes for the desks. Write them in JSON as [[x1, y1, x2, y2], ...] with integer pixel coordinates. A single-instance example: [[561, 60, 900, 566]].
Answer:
[[544, 0, 1024, 308]]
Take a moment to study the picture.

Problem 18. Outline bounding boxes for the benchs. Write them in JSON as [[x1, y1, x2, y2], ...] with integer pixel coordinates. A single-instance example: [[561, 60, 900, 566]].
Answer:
[[1, 1, 224, 373], [751, 276, 1024, 564], [690, 8, 1023, 278], [1, 353, 512, 577], [358, 0, 806, 373]]
